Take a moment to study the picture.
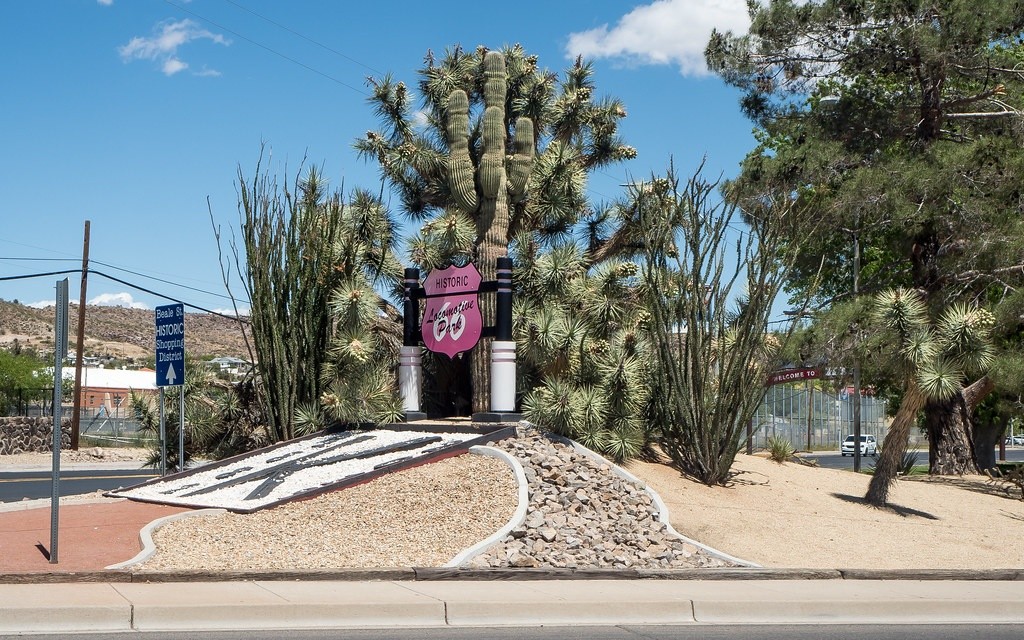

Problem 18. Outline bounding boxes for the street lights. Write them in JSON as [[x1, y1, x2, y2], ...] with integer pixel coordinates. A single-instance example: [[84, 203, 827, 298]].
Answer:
[[818, 95, 861, 473]]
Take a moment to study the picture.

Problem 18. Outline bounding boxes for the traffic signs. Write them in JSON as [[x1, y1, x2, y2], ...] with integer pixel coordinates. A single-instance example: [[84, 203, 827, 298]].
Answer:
[[154, 302, 185, 386]]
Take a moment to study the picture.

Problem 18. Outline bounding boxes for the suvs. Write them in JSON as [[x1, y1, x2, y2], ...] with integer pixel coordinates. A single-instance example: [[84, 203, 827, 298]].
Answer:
[[841, 434, 877, 457]]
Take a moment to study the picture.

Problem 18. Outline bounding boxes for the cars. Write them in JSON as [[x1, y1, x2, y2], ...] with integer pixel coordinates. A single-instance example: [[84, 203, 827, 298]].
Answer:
[[1005, 436, 1024, 446]]
